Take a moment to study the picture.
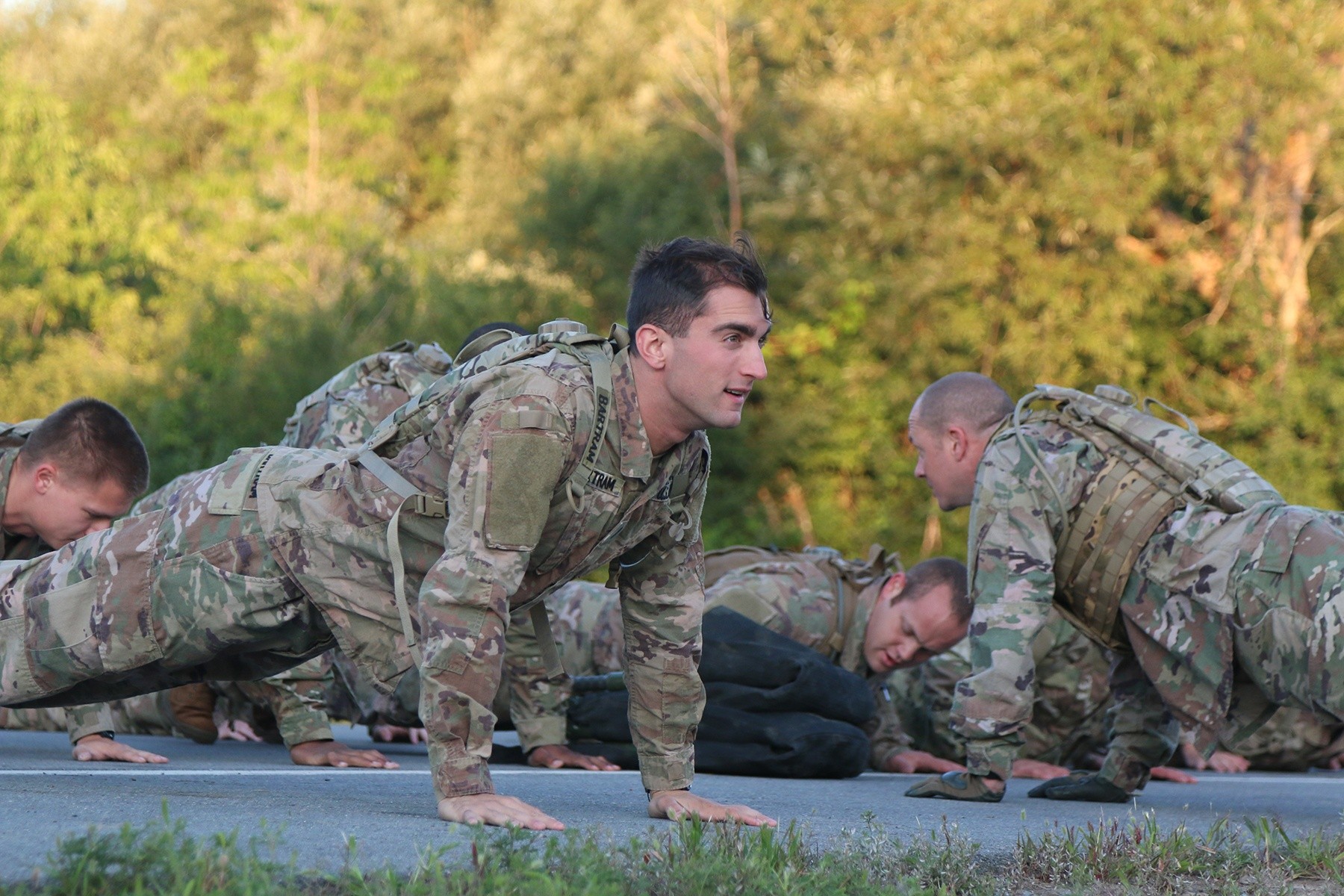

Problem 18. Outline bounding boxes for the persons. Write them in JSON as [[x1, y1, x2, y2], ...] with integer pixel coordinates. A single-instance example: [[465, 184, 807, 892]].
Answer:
[[0, 396, 1344, 788], [903, 370, 1344, 803], [0, 235, 780, 837]]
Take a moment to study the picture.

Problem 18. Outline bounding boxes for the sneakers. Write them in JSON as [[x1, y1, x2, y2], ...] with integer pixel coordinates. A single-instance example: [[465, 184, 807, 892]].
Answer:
[[157, 683, 220, 744]]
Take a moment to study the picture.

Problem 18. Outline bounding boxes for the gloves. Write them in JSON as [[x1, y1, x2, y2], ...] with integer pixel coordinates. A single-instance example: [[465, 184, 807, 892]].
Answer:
[[905, 769, 1005, 802], [1029, 772, 1130, 803]]
